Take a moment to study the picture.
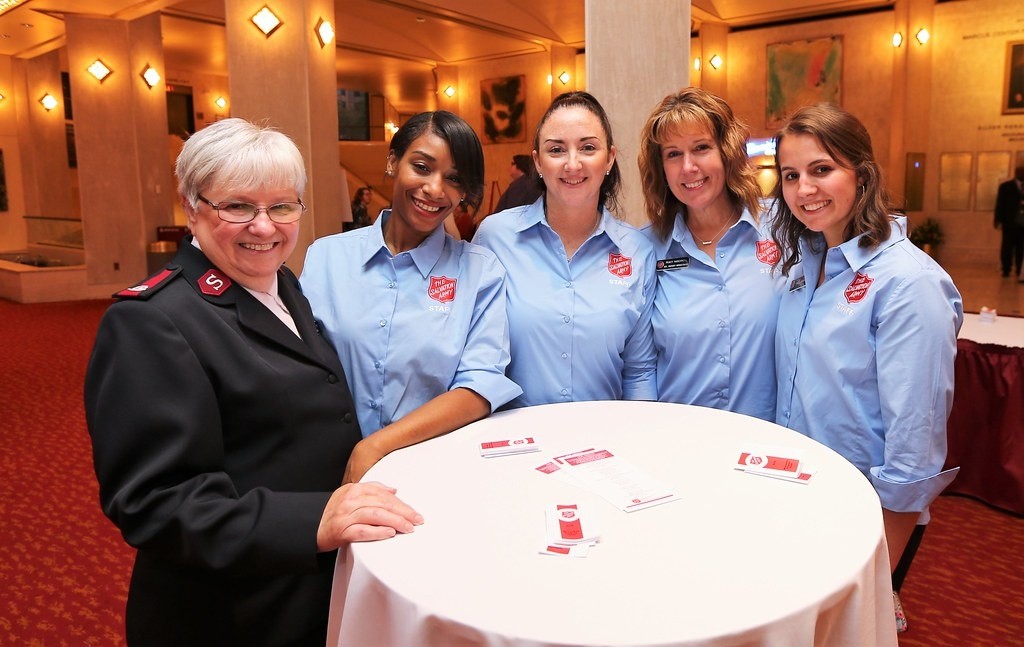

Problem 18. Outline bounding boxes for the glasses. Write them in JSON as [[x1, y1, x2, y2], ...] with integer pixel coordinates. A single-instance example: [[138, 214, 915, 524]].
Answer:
[[510, 162, 516, 168], [197, 193, 307, 224]]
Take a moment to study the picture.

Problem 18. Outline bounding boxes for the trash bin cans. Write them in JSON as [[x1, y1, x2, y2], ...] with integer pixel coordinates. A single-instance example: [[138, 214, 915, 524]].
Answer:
[[149, 241, 178, 274]]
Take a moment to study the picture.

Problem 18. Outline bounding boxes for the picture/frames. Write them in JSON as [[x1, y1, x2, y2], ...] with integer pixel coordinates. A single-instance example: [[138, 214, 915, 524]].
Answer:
[[765, 34, 844, 130], [1000, 40, 1024, 115]]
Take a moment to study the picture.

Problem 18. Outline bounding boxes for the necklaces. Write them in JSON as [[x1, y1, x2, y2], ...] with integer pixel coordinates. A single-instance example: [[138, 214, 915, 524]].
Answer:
[[687, 209, 735, 246], [568, 214, 600, 263]]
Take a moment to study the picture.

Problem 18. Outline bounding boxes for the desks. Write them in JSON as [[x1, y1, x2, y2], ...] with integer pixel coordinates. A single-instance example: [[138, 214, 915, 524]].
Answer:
[[349, 400, 884, 647]]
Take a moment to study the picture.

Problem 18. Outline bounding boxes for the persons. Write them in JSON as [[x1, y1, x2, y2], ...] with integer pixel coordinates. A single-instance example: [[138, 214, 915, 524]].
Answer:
[[771, 102, 963, 596], [637, 86, 802, 425], [342, 154, 540, 241], [83, 118, 423, 647], [470, 91, 656, 412], [297, 111, 523, 485]]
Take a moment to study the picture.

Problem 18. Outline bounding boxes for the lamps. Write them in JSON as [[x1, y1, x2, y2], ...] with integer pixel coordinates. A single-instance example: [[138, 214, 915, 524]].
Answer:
[[709, 55, 723, 69], [248, 4, 283, 39], [139, 63, 160, 90], [559, 71, 570, 84], [38, 93, 59, 113], [88, 58, 115, 85], [445, 86, 454, 97], [915, 29, 931, 45], [314, 17, 335, 49], [216, 97, 226, 108], [891, 32, 903, 47]]
